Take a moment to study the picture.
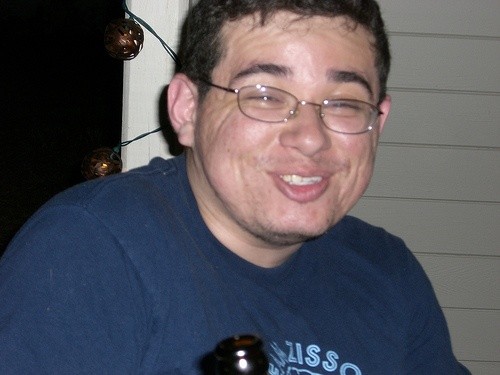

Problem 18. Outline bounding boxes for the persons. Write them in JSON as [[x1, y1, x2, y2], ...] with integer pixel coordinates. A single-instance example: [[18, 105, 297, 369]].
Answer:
[[0, 0, 471, 375]]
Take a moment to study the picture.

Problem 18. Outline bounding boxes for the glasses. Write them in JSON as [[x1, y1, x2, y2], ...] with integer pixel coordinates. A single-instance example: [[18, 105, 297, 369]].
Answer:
[[193, 76, 385, 135]]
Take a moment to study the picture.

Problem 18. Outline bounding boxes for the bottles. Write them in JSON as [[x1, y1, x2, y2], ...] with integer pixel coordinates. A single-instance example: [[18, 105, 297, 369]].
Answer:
[[215, 335, 271, 375]]
